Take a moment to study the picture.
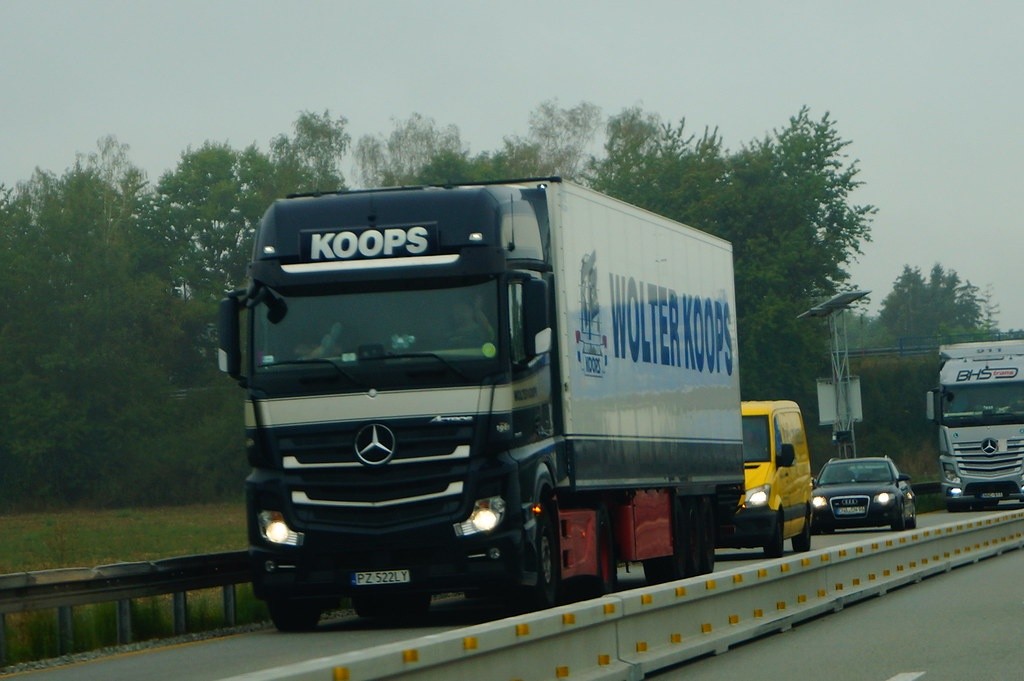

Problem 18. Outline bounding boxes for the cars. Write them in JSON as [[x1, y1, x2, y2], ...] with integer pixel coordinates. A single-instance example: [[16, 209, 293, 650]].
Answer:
[[810, 452, 917, 535]]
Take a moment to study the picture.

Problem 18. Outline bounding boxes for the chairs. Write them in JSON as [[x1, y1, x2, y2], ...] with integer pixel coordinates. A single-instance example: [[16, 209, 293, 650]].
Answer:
[[871, 466, 889, 481], [834, 469, 855, 483]]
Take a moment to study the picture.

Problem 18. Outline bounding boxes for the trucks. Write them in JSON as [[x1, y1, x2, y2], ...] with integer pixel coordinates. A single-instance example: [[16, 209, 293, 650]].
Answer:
[[924, 339, 1024, 513], [218, 175, 750, 633]]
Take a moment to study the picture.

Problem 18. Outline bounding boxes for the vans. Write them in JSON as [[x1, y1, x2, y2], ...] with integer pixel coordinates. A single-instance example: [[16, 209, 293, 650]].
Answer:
[[708, 399, 815, 561]]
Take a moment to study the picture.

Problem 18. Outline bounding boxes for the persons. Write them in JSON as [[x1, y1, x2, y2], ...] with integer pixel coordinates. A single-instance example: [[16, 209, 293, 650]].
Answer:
[[293, 314, 349, 360], [444, 293, 494, 348]]
